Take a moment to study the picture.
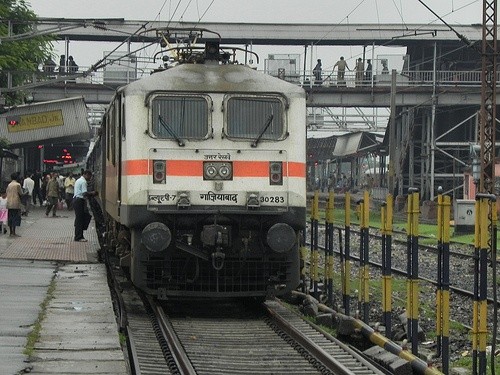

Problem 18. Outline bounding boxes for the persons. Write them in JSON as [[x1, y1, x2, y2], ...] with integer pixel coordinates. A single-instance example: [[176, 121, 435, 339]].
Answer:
[[73, 168, 96, 242], [313, 59, 322, 84], [46, 171, 61, 218], [353, 58, 389, 87], [6, 173, 23, 238], [307, 174, 374, 200], [22, 167, 76, 216], [42, 54, 79, 75], [0, 188, 8, 234], [333, 56, 351, 80]]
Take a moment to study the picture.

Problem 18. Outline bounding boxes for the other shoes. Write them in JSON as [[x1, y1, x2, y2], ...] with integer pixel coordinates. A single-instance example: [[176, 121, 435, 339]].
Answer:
[[75, 238, 88, 242], [4, 228, 9, 234]]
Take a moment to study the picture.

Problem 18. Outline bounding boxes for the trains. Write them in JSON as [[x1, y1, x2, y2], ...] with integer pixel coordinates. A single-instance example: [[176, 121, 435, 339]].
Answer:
[[83, 26, 306, 313]]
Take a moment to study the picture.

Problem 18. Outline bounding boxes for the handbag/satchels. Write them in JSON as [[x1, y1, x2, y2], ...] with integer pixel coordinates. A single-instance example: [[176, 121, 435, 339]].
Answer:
[[80, 213, 93, 230]]
